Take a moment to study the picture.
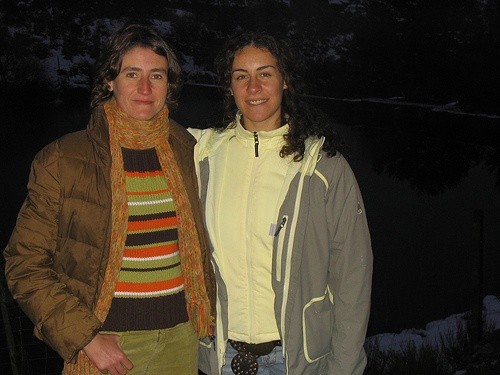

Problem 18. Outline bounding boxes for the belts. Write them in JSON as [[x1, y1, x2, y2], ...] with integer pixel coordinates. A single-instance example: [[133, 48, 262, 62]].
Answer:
[[227, 341, 282, 375]]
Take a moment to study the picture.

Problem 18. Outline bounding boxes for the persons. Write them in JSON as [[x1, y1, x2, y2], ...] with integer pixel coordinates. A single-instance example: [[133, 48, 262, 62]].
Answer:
[[183, 33, 374, 374], [1, 26, 220, 375]]
